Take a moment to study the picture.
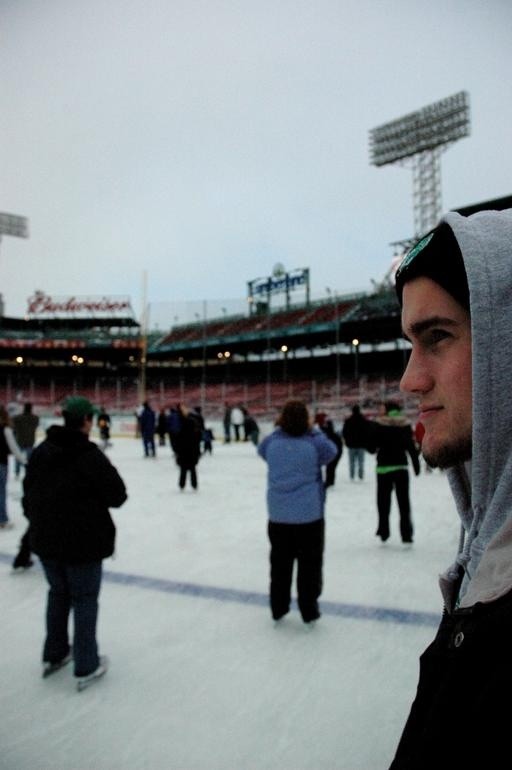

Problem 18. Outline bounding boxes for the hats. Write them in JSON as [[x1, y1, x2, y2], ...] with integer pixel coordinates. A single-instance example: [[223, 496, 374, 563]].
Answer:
[[394, 224, 473, 317], [62, 396, 100, 414]]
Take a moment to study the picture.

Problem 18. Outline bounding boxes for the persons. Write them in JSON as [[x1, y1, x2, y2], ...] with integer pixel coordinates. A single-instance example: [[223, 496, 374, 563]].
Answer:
[[367, 401, 421, 549], [98, 400, 260, 489], [1, 401, 62, 573], [385, 206, 511, 770], [314, 404, 435, 488], [21, 396, 128, 690], [259, 399, 337, 628], [1, 301, 424, 425]]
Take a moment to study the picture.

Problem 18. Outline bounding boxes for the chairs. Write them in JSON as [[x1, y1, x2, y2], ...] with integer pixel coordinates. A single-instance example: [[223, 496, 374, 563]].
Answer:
[[0, 328, 153, 351], [160, 301, 349, 344]]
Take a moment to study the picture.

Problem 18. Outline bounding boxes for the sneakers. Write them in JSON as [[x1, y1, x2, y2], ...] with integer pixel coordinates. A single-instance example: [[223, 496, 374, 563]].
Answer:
[[72, 662, 107, 682], [13, 558, 34, 570], [45, 646, 73, 667]]
